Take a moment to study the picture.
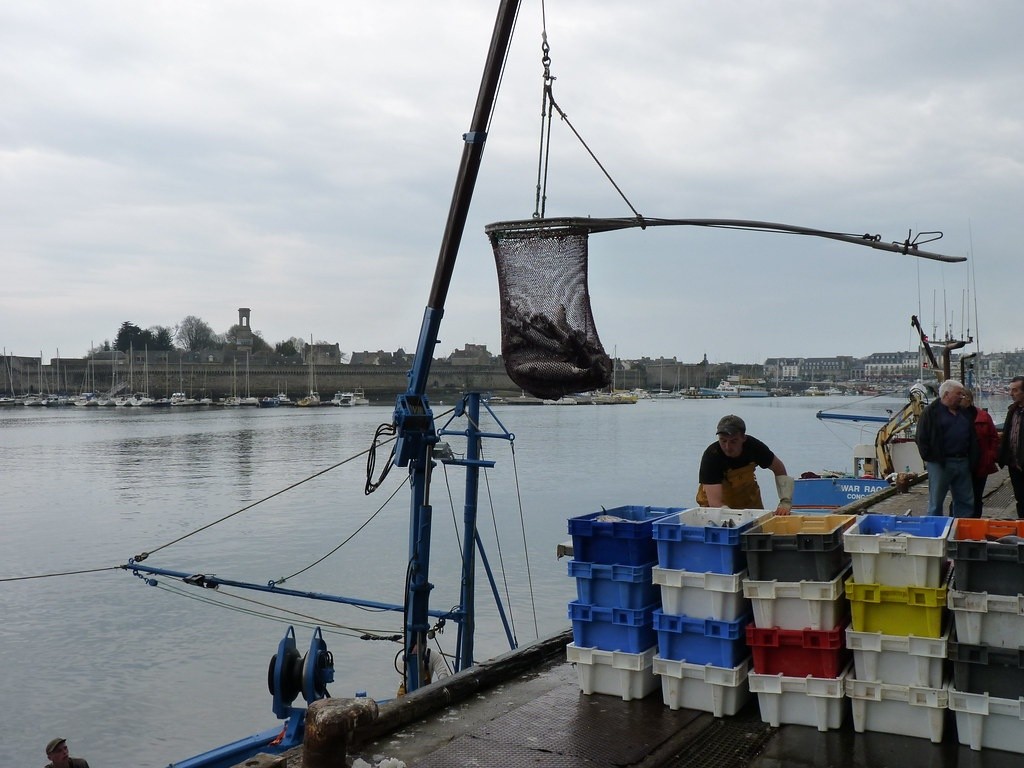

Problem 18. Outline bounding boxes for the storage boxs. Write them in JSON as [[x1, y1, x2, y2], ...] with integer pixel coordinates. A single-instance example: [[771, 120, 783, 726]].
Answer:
[[566, 503, 1024, 752]]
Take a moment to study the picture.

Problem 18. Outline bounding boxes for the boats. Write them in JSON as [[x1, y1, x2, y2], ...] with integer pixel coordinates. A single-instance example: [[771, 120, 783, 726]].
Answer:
[[542, 342, 879, 405]]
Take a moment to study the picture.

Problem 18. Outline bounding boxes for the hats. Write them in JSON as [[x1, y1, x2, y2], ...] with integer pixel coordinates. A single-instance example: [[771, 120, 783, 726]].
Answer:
[[46, 738, 66, 754], [715, 415, 746, 436]]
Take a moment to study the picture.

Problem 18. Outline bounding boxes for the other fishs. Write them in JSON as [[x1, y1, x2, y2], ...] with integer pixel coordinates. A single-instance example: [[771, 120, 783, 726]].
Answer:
[[501, 304, 611, 399], [590, 504, 736, 528]]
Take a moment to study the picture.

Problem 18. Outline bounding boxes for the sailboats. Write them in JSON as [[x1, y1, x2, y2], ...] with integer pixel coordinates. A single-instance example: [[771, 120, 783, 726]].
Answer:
[[886, 215, 1005, 476], [0, 333, 371, 407]]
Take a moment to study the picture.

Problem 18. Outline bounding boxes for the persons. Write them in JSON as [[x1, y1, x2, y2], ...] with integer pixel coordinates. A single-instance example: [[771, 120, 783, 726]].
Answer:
[[915, 379, 976, 517], [948, 390, 999, 518], [43, 737, 90, 768], [696, 415, 795, 516], [397, 640, 448, 698], [1000, 375, 1024, 519]]
